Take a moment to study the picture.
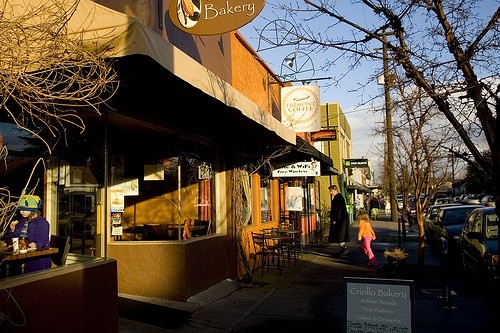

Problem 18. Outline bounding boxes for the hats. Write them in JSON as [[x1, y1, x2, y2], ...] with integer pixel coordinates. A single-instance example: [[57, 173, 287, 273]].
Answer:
[[18, 195, 42, 211]]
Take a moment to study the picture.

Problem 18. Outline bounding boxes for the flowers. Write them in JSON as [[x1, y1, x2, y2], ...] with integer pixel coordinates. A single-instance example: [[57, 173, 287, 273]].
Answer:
[[383, 247, 408, 260]]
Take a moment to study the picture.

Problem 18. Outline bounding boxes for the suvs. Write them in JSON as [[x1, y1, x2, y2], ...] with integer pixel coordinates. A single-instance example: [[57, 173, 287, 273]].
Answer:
[[461, 196, 480, 205], [481, 196, 496, 206], [459, 194, 469, 204]]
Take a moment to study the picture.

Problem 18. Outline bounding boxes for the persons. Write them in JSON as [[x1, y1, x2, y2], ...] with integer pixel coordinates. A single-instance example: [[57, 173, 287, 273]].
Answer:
[[357, 211, 378, 265], [9, 194, 51, 274], [328, 185, 350, 249], [363, 195, 385, 221]]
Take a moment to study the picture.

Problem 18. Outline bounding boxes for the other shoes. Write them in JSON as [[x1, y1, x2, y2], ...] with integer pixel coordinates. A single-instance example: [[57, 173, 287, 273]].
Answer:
[[335, 245, 347, 254], [368, 263, 378, 266]]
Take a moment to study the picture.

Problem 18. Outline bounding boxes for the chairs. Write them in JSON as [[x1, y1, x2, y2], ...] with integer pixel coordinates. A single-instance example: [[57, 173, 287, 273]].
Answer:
[[50, 235, 71, 266], [252, 224, 303, 278], [142, 223, 169, 240], [192, 219, 211, 237]]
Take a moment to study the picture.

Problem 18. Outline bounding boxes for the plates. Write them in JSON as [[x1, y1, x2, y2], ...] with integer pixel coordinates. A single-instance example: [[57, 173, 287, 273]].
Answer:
[[27, 247, 37, 251]]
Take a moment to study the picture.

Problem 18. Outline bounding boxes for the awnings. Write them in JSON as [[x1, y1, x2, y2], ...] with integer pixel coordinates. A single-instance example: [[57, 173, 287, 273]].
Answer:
[[0, 0, 340, 174]]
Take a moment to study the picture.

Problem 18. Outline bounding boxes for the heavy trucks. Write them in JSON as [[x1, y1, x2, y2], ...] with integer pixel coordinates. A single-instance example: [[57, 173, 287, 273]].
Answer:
[[432, 191, 452, 204]]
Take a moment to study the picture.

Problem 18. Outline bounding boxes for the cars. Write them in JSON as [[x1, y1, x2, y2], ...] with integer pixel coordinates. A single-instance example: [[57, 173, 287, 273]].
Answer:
[[424, 204, 462, 233], [396, 195, 432, 218], [459, 207, 500, 281], [431, 205, 486, 256]]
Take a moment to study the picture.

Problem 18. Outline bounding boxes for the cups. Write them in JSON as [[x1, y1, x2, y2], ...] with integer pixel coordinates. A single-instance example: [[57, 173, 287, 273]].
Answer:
[[18, 240, 26, 250], [12, 237, 19, 252]]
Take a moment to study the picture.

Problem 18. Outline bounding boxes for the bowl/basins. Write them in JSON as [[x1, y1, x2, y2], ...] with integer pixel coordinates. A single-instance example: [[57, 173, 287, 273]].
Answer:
[[20, 250, 27, 254]]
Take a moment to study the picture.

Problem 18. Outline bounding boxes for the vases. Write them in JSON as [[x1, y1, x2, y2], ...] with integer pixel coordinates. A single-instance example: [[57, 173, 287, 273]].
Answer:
[[388, 256, 407, 268]]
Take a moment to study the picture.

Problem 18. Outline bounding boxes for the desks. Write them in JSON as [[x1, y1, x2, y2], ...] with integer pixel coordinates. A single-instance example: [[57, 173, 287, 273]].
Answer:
[[134, 224, 205, 230], [0, 248, 59, 277], [255, 235, 291, 239], [275, 230, 303, 234]]
